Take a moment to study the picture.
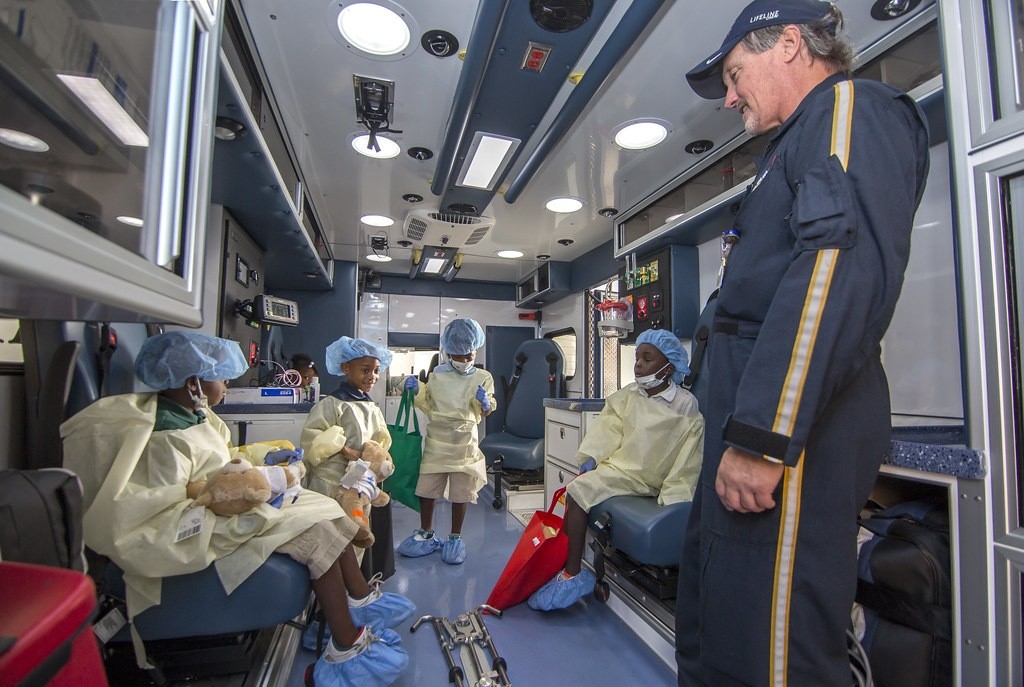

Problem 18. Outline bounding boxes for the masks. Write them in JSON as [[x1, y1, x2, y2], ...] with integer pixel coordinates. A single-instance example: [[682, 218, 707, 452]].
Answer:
[[447, 353, 475, 372], [187, 377, 232, 444], [635, 362, 672, 390]]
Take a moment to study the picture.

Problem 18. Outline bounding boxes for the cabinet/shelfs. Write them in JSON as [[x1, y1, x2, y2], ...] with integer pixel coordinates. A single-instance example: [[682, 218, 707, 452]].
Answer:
[[542, 398, 605, 517]]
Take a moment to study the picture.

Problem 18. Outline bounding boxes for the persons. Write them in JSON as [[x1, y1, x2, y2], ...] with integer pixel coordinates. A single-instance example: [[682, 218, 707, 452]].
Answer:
[[101, 329, 415, 687], [673, 0, 928, 687], [528, 330, 705, 611], [398, 317, 497, 565]]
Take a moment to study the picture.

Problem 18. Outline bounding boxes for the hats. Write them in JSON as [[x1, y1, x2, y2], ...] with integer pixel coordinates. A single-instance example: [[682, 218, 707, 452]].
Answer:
[[686, 0, 832, 100]]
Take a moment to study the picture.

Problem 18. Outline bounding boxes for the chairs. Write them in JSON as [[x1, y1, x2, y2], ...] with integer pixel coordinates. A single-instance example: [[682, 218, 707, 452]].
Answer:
[[34, 338, 311, 642], [588, 495, 693, 599], [478, 338, 566, 469]]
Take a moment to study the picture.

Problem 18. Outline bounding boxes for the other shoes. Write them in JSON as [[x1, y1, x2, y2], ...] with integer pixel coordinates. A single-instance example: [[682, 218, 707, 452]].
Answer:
[[348, 572, 385, 608], [323, 625, 387, 663], [413, 528, 461, 541]]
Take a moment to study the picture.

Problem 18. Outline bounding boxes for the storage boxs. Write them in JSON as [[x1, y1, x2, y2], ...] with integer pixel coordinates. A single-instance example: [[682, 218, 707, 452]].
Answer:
[[223, 388, 306, 402]]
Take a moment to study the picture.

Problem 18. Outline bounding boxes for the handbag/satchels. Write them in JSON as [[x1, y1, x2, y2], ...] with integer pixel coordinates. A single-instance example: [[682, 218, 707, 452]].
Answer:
[[378, 386, 423, 513], [483, 482, 567, 614]]
